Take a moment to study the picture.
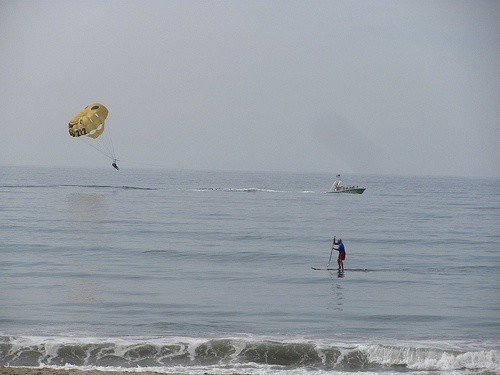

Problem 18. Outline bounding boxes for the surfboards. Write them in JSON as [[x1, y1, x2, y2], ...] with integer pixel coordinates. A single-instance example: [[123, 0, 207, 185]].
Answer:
[[310, 267, 369, 272]]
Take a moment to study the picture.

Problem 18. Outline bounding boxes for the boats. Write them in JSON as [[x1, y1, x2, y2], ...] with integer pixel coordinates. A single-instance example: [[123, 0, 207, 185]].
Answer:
[[326, 184, 368, 195]]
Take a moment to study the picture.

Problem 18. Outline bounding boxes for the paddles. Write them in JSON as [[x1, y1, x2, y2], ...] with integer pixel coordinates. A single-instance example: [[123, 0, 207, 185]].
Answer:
[[327, 235, 336, 264]]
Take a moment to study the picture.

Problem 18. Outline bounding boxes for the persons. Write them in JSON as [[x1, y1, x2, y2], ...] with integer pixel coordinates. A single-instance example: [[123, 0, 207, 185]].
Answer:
[[332, 237, 346, 278], [112, 162, 119, 170]]
